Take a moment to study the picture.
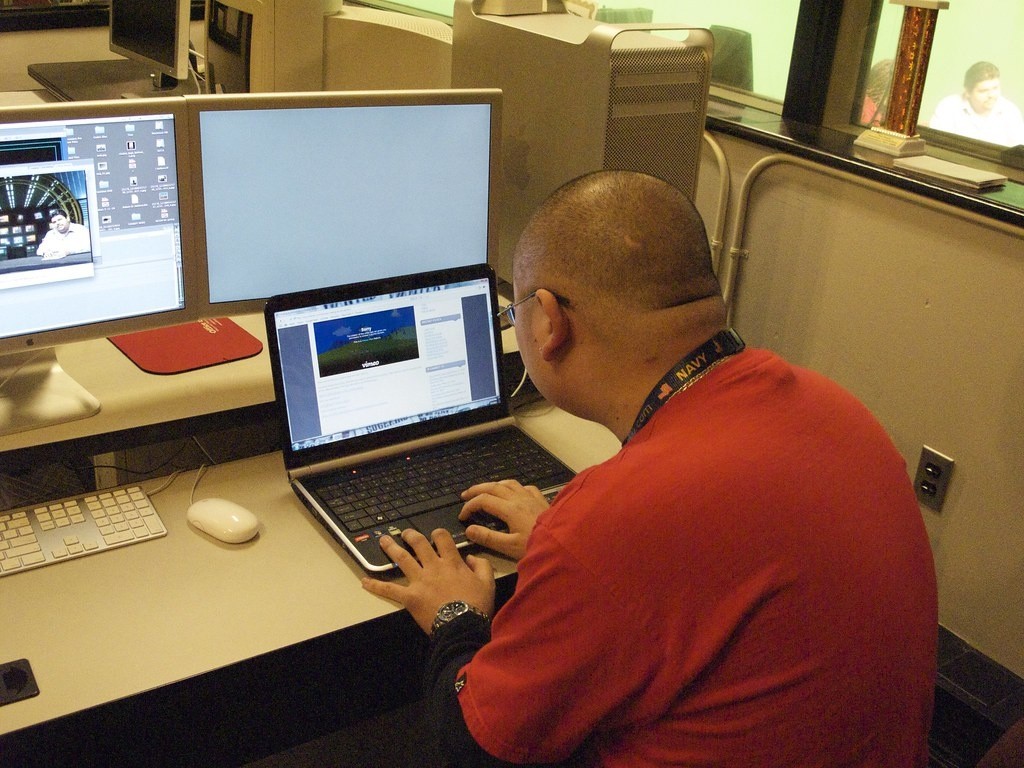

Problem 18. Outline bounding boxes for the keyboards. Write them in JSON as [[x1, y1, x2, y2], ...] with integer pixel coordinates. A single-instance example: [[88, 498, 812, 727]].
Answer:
[[0, 486, 168, 577]]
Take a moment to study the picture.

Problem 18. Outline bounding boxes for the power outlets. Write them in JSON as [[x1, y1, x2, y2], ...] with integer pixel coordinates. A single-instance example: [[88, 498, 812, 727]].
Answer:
[[912, 444, 955, 512]]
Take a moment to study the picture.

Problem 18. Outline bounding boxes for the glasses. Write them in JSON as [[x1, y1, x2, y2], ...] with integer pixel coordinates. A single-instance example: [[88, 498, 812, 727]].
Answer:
[[497, 288, 571, 328]]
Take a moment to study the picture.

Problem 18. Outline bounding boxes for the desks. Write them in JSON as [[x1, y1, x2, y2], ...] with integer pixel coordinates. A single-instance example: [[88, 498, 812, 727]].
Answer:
[[0, 390, 622, 768], [0, 294, 519, 490]]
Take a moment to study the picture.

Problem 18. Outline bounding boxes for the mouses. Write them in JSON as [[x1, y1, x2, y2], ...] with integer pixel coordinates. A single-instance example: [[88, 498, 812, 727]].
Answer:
[[187, 498, 261, 543]]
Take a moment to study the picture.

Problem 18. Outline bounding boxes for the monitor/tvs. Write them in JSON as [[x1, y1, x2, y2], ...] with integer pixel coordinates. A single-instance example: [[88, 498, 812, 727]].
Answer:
[[0, 0, 503, 437]]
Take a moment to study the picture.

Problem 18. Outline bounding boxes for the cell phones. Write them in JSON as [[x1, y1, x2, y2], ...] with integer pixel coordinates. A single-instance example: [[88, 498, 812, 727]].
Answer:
[[0, 659, 40, 707]]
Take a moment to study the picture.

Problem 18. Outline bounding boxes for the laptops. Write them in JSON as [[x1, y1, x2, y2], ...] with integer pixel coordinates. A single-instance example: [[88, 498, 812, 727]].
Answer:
[[264, 263, 580, 578]]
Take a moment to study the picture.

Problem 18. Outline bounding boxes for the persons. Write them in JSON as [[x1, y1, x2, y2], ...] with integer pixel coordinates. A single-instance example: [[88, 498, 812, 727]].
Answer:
[[860, 60, 896, 127], [36, 209, 93, 257], [361, 165, 938, 768], [929, 60, 1024, 148]]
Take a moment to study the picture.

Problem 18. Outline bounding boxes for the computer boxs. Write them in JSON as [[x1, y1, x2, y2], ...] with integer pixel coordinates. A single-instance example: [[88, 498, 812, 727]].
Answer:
[[453, 0, 714, 296]]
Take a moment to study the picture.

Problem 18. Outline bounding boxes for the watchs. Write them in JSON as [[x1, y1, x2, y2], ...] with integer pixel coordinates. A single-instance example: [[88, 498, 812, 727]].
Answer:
[[428, 600, 493, 635]]
